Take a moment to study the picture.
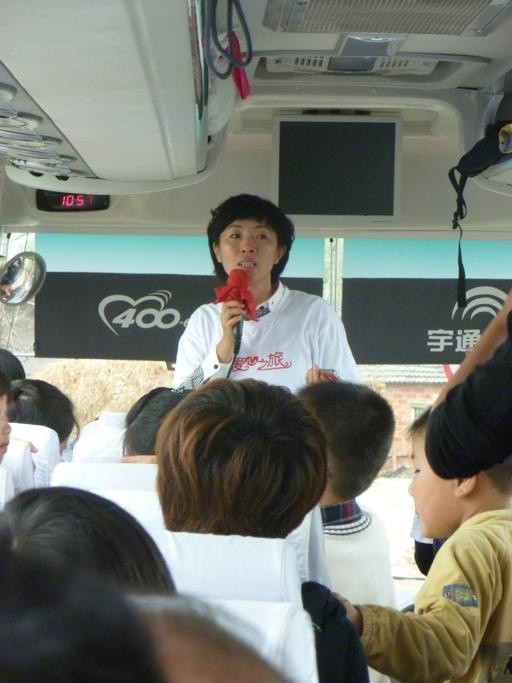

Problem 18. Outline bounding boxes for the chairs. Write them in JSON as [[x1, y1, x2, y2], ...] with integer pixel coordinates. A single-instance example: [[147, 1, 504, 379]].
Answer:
[[0, 413, 332, 683]]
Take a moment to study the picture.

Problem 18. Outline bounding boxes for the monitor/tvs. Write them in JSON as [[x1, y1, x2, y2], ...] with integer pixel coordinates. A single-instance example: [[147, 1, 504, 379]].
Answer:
[[271, 114, 402, 227]]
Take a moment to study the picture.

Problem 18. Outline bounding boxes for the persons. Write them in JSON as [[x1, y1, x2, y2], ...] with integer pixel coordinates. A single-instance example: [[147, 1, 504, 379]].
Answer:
[[120, 386, 199, 465], [1, 348, 80, 472], [1, 523, 289, 682], [1, 486, 180, 597], [424, 283, 512, 479], [331, 404, 512, 683], [172, 193, 364, 391], [294, 381, 396, 682], [153, 376, 369, 683]]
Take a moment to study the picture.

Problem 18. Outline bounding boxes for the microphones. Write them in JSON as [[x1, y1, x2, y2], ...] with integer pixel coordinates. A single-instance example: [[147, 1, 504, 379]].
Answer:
[[212, 270, 261, 355]]
